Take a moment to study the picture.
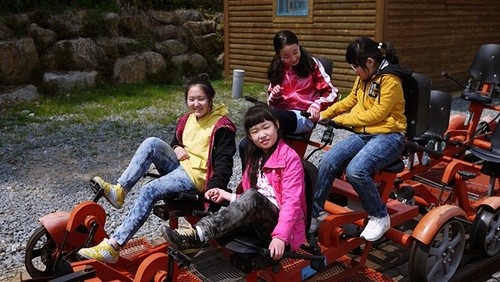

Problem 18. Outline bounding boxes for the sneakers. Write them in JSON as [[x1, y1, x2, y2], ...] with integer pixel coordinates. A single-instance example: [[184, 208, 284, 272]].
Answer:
[[77, 238, 119, 264], [308, 217, 319, 234], [92, 176, 124, 209], [161, 226, 207, 255], [359, 214, 390, 242]]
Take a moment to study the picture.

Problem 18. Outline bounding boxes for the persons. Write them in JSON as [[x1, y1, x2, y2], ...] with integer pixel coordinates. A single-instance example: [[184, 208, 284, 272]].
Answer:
[[309, 37, 407, 242], [161, 104, 306, 267], [239, 30, 339, 175], [78, 74, 237, 263]]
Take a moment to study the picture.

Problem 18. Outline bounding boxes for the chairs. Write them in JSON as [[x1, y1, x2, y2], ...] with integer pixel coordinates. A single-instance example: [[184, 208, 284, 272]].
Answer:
[[283, 56, 333, 140], [373, 72, 431, 174], [469, 120, 500, 163], [412, 90, 452, 159], [214, 160, 318, 258], [461, 44, 500, 104]]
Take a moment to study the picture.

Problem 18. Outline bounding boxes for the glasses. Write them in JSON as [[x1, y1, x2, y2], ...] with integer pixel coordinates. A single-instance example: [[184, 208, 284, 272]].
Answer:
[[350, 62, 367, 71]]
